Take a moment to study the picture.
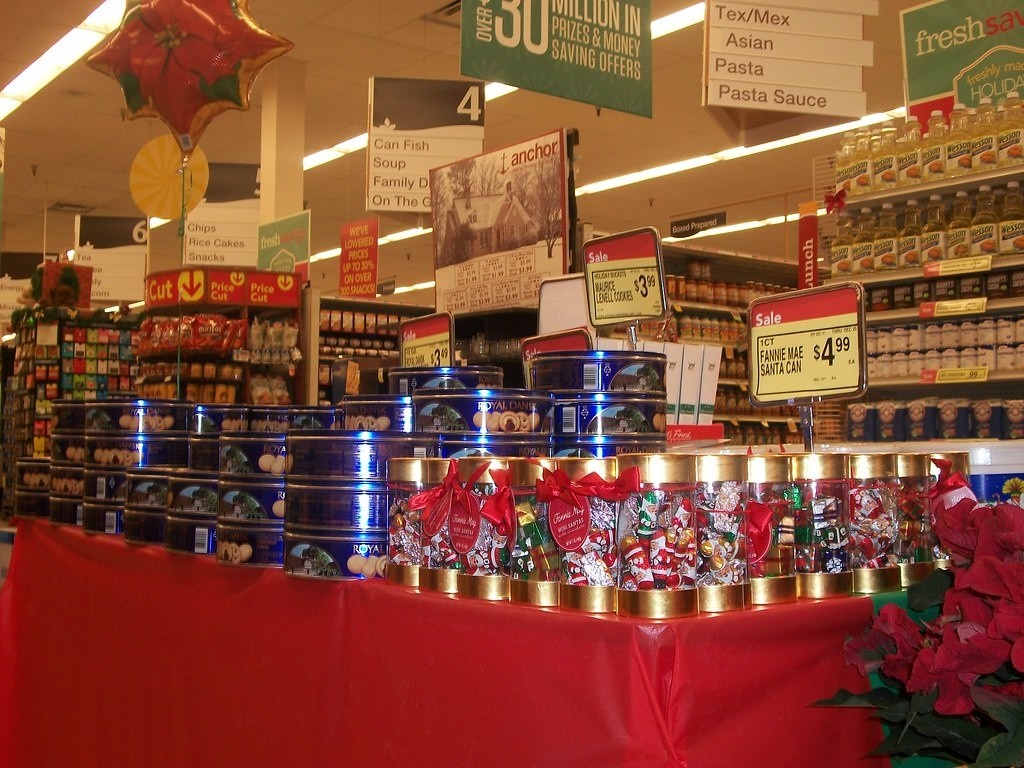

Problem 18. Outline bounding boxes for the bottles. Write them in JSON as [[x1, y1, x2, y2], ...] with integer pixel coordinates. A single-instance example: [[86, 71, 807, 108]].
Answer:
[[831, 91, 1024, 277]]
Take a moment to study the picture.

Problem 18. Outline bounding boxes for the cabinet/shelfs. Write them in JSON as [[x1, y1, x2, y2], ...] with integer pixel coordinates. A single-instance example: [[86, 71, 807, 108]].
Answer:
[[0, 123, 1024, 541]]
